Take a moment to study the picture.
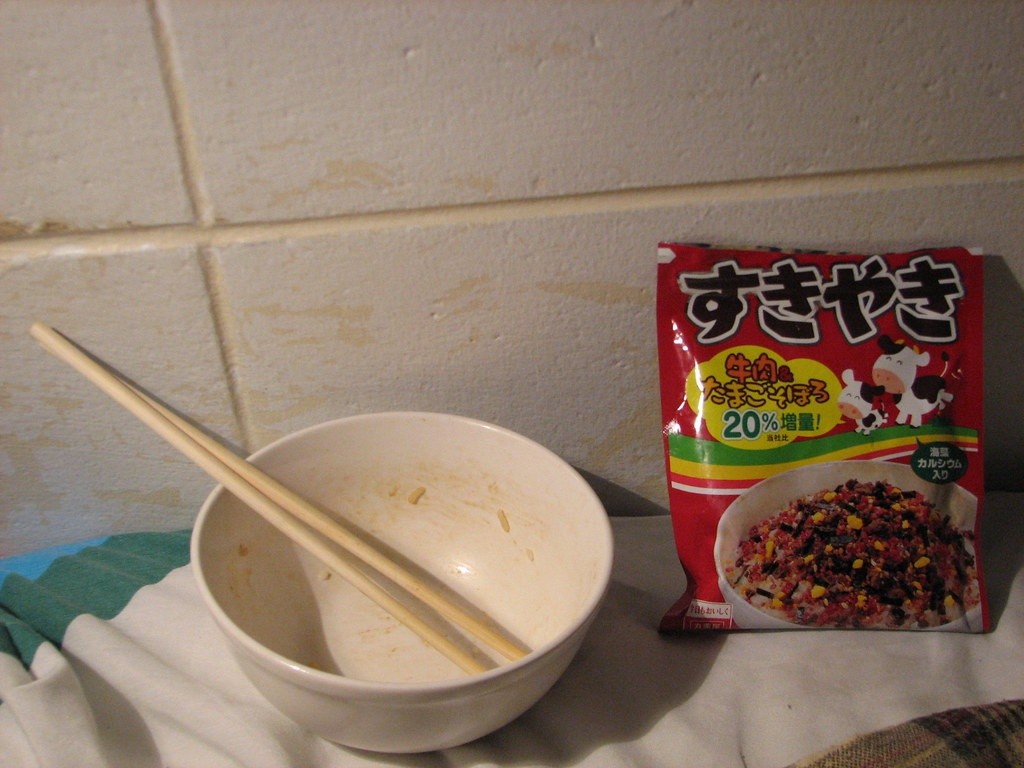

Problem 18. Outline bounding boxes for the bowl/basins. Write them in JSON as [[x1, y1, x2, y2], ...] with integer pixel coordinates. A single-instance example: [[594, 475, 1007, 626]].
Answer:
[[190, 412, 615, 754], [715, 460, 982, 631]]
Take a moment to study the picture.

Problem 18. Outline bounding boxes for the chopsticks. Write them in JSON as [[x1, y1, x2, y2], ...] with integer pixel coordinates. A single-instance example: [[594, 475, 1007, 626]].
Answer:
[[29, 320, 531, 679]]
